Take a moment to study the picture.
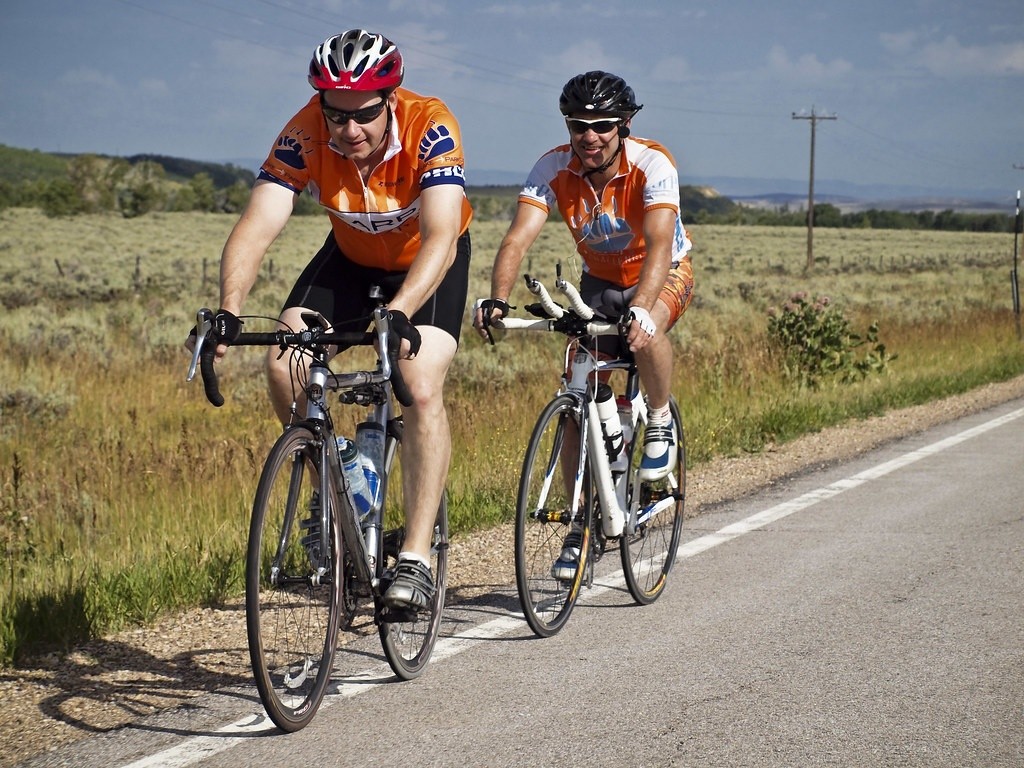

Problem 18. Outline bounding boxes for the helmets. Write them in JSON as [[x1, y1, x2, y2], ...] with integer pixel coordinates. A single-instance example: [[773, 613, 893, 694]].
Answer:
[[308, 29, 405, 92], [559, 70, 641, 120]]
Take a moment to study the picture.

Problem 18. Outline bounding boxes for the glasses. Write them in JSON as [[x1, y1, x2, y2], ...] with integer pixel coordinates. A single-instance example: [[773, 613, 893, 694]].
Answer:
[[565, 117, 623, 134], [321, 91, 388, 124]]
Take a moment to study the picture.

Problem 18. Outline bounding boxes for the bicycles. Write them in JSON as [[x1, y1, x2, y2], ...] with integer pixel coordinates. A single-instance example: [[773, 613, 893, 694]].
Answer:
[[188, 283, 450, 735], [481, 264, 690, 641]]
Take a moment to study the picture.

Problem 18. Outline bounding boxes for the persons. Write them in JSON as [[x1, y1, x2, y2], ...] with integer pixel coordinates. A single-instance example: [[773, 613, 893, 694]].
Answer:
[[469, 70, 696, 584], [184, 25, 478, 607]]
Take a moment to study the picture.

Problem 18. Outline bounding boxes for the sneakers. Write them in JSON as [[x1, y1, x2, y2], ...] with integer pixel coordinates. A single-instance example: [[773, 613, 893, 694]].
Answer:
[[299, 489, 332, 568], [383, 558, 433, 606], [551, 528, 589, 580], [637, 418, 678, 482]]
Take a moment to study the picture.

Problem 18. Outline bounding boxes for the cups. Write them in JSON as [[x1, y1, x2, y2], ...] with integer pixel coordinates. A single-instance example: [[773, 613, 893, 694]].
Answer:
[[616, 394, 634, 455], [337, 436, 371, 517], [355, 412, 385, 510], [592, 382, 626, 458]]
